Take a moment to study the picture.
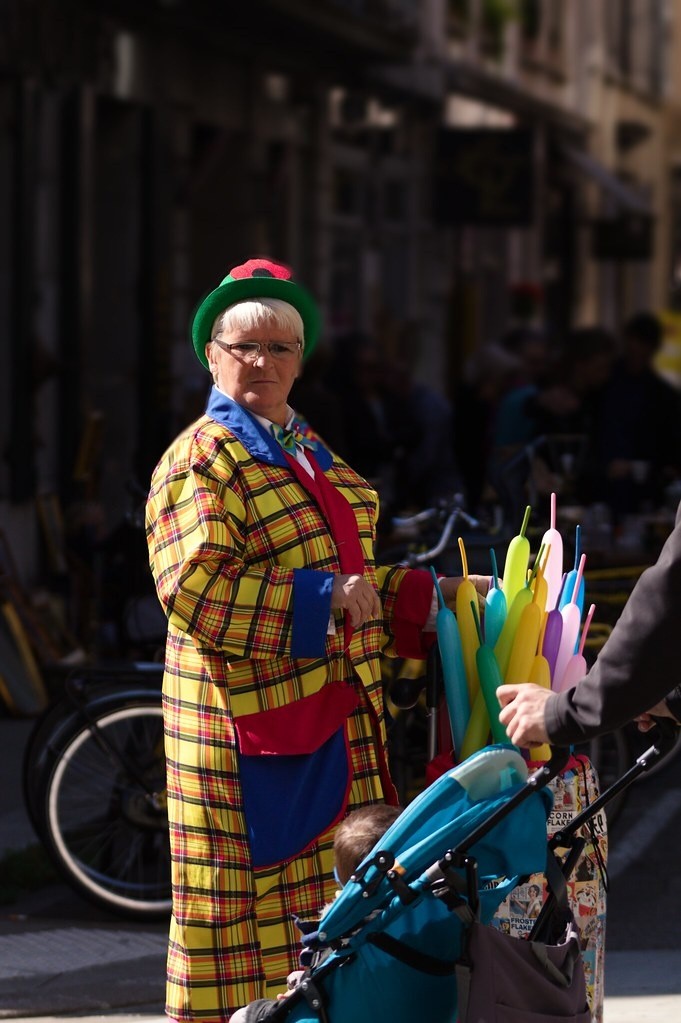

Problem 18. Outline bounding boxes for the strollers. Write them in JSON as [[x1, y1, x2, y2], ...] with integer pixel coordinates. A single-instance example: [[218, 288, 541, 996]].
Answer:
[[185, 716, 679, 1022]]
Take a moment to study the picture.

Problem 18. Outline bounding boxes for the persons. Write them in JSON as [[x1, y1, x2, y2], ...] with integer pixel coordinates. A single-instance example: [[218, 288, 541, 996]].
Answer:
[[143, 257, 503, 1023], [228, 803, 403, 1023], [293, 310, 681, 537], [496, 503, 681, 749]]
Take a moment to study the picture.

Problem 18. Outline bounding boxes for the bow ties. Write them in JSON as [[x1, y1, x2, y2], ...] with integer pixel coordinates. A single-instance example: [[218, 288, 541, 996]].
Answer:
[[271, 421, 317, 457]]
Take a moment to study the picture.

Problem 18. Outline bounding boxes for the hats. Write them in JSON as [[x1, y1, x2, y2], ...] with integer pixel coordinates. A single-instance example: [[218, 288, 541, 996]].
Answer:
[[191, 260, 320, 370]]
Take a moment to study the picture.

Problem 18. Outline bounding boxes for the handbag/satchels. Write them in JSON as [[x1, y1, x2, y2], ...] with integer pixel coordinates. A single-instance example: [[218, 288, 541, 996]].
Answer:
[[456, 924, 592, 1022]]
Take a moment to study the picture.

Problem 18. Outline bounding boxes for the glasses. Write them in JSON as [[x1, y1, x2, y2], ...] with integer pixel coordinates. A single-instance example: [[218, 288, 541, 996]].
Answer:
[[213, 336, 301, 361]]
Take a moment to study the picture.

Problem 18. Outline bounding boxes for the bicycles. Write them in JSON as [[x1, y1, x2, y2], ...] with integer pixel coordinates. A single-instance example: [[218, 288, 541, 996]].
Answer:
[[19, 489, 484, 921]]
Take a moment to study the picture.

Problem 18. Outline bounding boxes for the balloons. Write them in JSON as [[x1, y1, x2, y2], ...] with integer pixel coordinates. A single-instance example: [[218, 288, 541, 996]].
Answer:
[[429, 493, 595, 762]]
